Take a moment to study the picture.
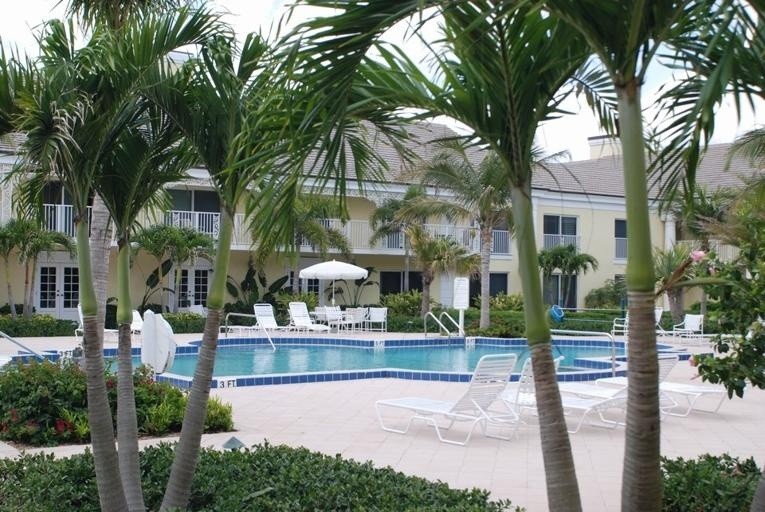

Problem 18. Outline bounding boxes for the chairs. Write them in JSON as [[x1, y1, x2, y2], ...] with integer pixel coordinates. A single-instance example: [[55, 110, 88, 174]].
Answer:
[[179, 218, 213, 235], [376, 353, 728, 447], [219, 301, 388, 336], [74, 303, 144, 347], [655, 307, 704, 344], [612, 305, 628, 341]]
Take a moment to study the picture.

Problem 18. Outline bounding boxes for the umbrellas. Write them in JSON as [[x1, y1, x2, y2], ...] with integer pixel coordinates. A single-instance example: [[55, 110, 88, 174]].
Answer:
[[299, 259, 368, 306]]
